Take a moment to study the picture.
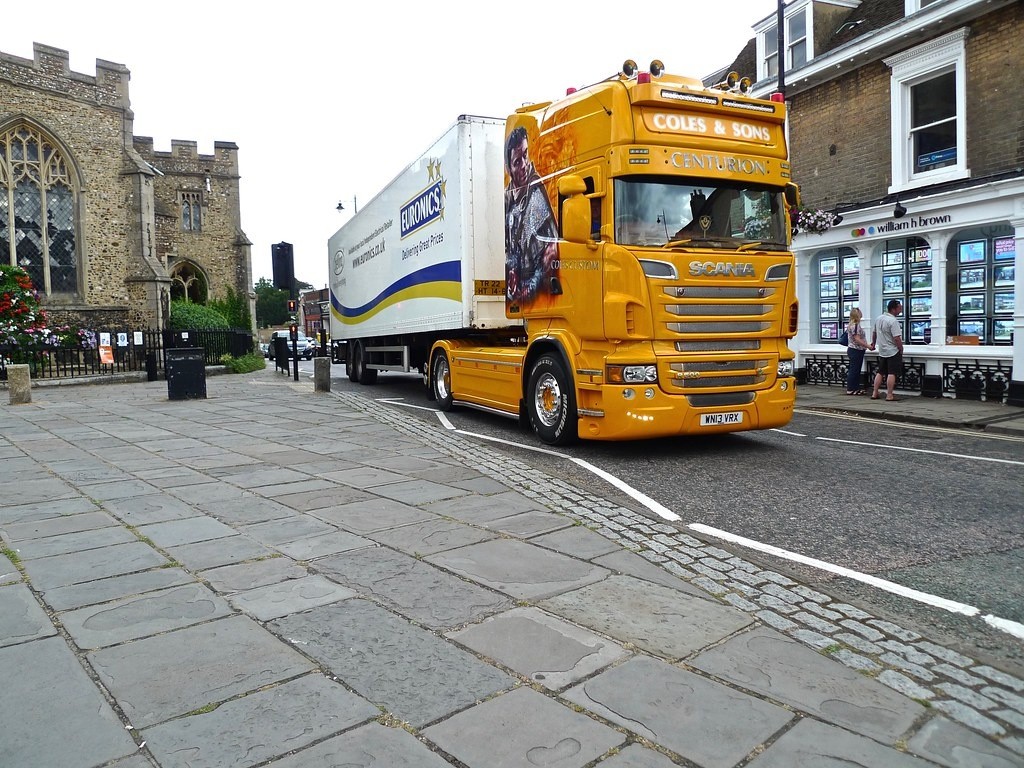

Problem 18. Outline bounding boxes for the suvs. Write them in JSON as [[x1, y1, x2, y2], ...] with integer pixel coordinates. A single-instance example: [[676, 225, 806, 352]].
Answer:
[[268, 330, 312, 361]]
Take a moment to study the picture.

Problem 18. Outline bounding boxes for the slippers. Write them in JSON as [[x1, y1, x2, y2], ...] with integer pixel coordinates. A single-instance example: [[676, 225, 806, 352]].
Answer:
[[886, 395, 904, 401], [846, 388, 868, 396], [870, 392, 880, 400]]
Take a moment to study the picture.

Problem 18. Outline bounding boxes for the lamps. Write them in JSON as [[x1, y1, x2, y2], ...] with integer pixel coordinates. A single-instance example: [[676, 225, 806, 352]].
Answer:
[[893, 193, 907, 218], [833, 202, 854, 225]]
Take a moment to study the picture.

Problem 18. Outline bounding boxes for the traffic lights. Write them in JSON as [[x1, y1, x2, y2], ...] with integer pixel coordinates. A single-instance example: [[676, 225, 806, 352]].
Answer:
[[287, 300, 298, 314], [289, 324, 298, 341]]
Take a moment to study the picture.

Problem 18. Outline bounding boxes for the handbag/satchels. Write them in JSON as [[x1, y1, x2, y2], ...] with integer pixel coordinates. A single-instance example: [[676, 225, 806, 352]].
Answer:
[[838, 329, 848, 346]]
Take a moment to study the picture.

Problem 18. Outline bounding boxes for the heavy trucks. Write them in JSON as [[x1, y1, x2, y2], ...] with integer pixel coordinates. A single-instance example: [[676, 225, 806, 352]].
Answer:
[[325, 57, 804, 449]]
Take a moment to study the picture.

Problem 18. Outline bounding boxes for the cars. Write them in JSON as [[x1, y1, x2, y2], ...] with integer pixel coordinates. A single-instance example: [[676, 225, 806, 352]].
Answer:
[[307, 336, 347, 358], [258, 343, 270, 358]]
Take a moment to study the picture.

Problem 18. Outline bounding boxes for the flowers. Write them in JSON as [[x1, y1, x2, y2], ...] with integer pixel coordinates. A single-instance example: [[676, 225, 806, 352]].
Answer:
[[740, 201, 836, 239]]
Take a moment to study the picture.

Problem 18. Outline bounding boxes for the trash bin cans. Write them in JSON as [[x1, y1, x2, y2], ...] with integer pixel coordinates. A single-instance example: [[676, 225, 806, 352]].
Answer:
[[165, 347, 207, 401]]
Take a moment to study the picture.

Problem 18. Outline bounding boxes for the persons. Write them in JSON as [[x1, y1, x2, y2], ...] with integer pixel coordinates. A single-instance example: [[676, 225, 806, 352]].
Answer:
[[847, 308, 876, 394], [871, 300, 903, 401], [504, 126, 561, 309]]
